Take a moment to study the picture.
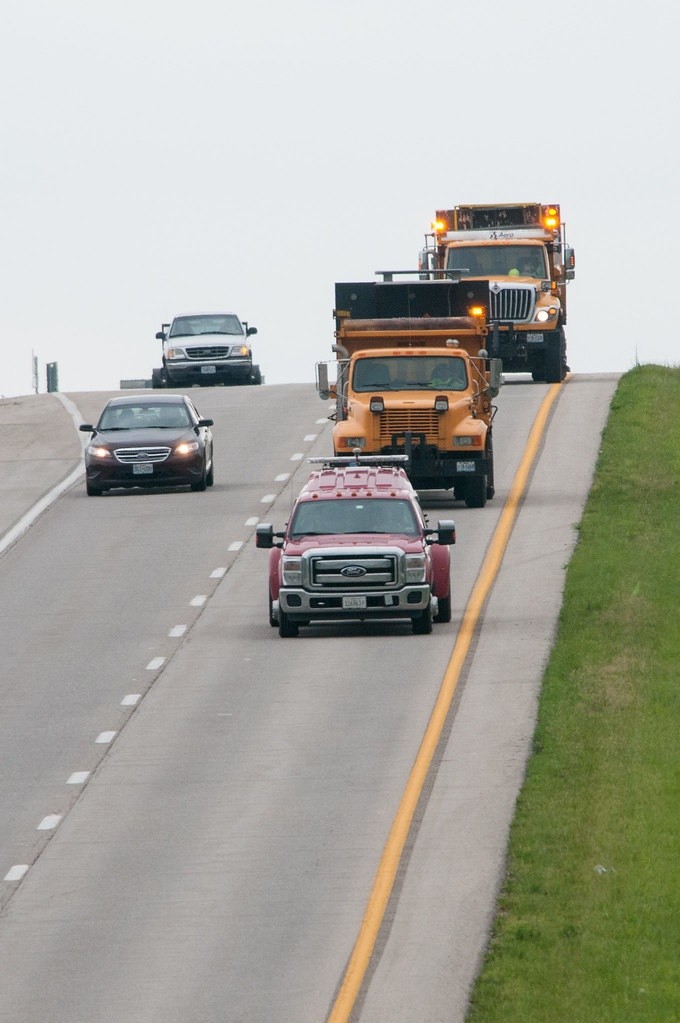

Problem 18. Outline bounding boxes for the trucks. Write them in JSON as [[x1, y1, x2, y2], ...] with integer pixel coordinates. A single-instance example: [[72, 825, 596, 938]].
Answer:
[[418, 201, 576, 385], [316, 265, 504, 507]]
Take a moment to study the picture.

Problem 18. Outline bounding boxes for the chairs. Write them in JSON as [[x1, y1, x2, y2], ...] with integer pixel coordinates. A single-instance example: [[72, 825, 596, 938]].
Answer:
[[363, 364, 390, 385], [431, 363, 458, 381], [119, 409, 135, 424], [158, 408, 187, 427]]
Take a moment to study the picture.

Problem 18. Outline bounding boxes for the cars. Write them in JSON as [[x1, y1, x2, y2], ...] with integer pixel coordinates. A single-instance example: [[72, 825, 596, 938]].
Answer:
[[257, 455, 458, 638], [78, 395, 215, 496], [155, 314, 258, 388]]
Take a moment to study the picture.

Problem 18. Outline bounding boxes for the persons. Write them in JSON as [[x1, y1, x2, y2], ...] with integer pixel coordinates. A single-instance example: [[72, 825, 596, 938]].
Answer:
[[524, 250, 543, 277], [429, 364, 464, 386]]
